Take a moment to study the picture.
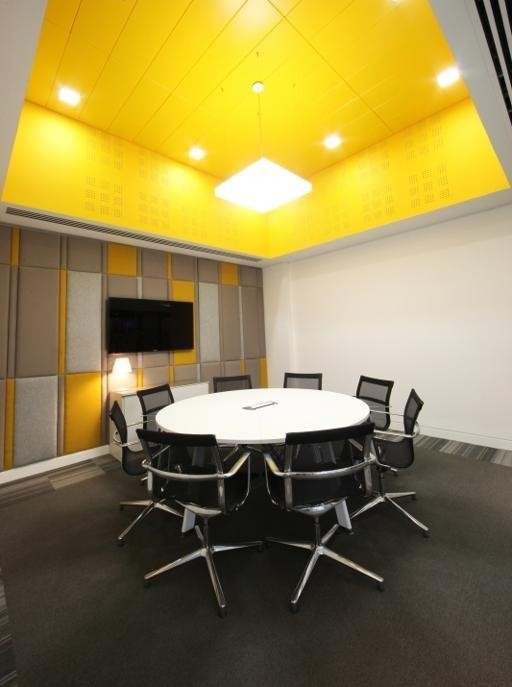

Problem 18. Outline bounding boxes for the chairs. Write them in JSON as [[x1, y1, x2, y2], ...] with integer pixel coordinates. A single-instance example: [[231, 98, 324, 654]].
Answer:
[[109, 400, 187, 545], [211, 372, 255, 390], [260, 421, 386, 611], [135, 384, 180, 434], [344, 385, 431, 535], [282, 372, 323, 392], [116, 425, 253, 615], [354, 374, 391, 433]]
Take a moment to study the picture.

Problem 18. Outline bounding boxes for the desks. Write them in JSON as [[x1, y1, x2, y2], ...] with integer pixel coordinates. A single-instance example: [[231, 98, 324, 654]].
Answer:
[[159, 384, 373, 501]]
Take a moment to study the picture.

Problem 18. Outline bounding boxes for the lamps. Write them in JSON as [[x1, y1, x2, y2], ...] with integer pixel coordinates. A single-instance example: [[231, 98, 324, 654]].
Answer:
[[214, 81, 313, 213], [111, 358, 132, 392]]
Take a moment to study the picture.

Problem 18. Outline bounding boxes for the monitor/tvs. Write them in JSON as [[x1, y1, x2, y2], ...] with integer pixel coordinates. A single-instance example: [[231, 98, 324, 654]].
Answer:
[[105, 296, 194, 354]]
